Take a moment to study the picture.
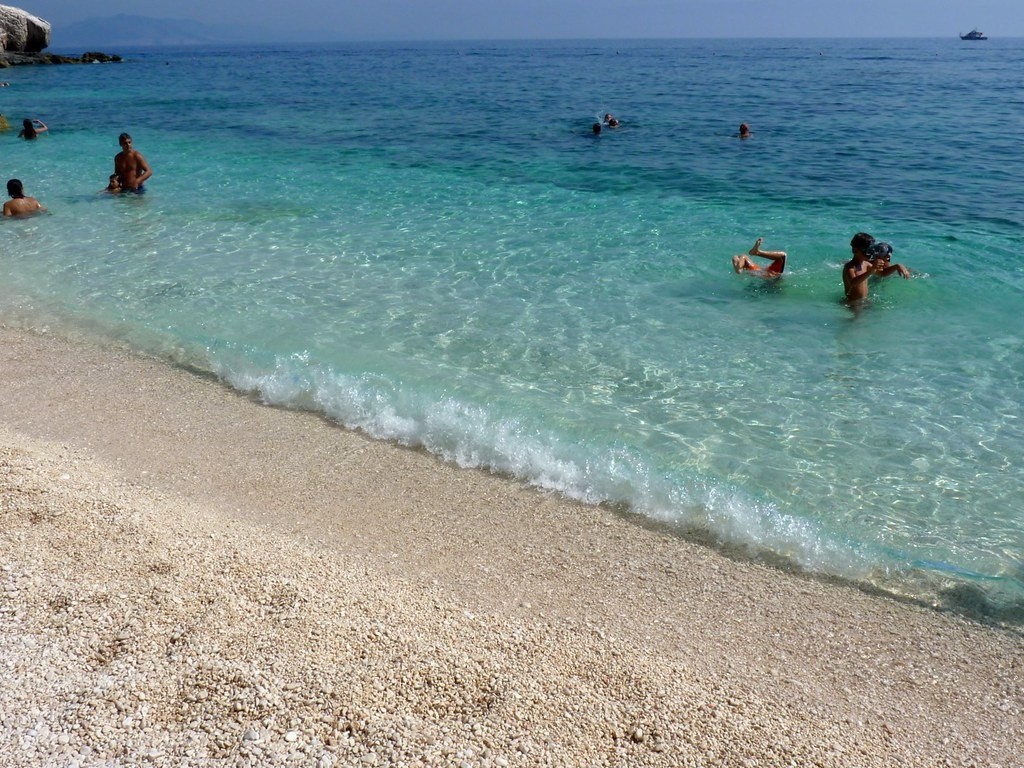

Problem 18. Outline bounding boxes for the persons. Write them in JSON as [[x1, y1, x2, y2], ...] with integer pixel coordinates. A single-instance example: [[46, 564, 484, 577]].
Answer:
[[732, 238, 786, 273], [604, 114, 612, 123], [609, 119, 620, 128], [843, 233, 910, 298], [3, 179, 40, 215], [18, 119, 48, 138], [871, 243, 892, 267], [114, 133, 152, 189], [109, 174, 121, 190], [740, 123, 749, 137], [593, 123, 601, 134]]
